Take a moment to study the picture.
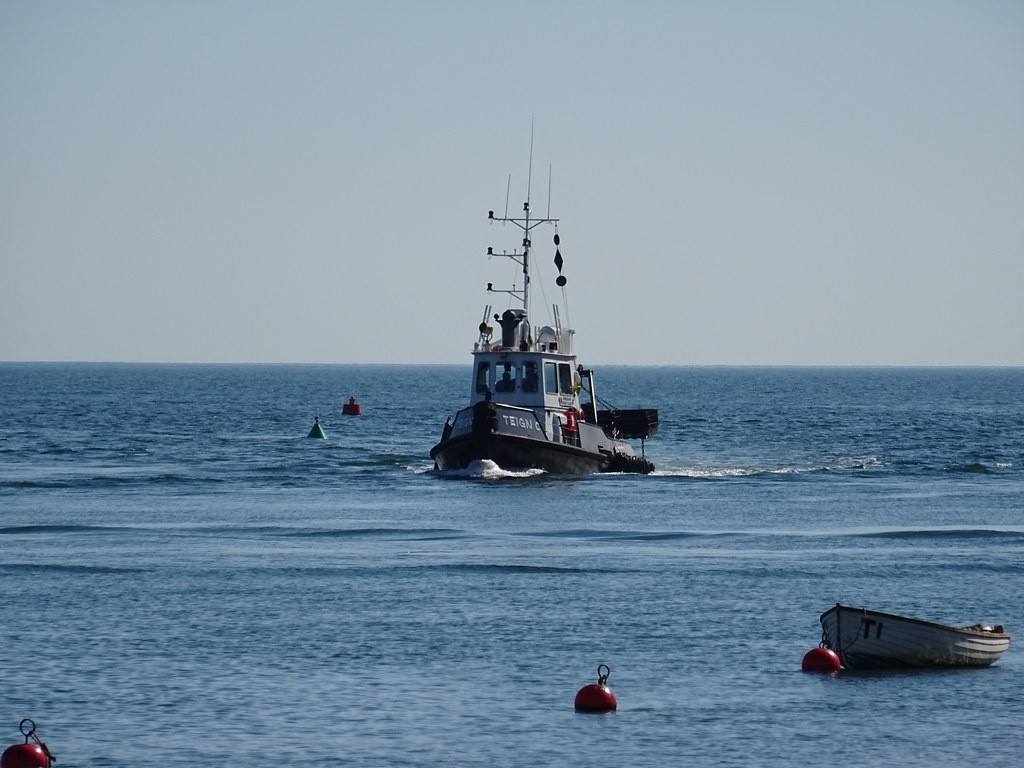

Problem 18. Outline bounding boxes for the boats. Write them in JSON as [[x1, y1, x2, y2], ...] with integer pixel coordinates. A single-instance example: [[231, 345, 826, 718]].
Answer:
[[820, 602, 1011, 673], [584, 408, 660, 440], [430, 113, 661, 479]]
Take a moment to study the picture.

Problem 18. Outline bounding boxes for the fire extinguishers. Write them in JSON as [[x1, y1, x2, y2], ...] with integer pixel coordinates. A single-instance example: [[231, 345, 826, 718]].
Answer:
[[567, 406, 577, 432]]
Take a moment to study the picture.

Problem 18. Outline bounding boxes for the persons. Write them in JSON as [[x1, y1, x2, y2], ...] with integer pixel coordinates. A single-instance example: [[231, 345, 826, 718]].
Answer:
[[494, 372, 516, 393]]
